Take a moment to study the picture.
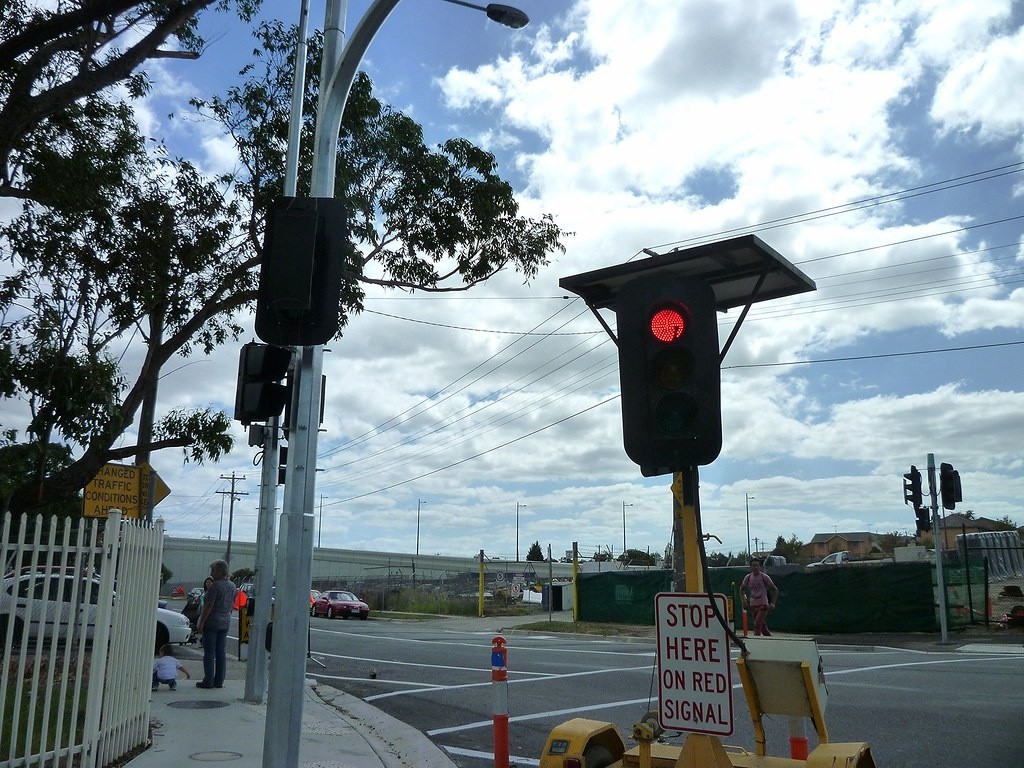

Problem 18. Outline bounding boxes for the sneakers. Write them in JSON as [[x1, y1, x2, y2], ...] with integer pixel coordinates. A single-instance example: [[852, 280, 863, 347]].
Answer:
[[196, 681, 214, 688], [170, 685, 178, 690], [214, 681, 223, 688], [152, 686, 158, 690]]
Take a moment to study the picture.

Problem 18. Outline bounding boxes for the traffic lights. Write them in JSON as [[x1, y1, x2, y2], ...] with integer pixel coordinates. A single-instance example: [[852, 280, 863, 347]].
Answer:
[[234, 342, 293, 422], [904, 465, 922, 506], [916, 508, 930, 532], [941, 463, 962, 510], [615, 277, 722, 477]]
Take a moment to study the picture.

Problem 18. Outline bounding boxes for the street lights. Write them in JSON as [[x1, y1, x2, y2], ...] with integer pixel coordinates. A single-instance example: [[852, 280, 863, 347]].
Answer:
[[417, 499, 426, 555], [517, 502, 526, 561], [746, 493, 755, 556], [318, 494, 328, 547], [623, 501, 634, 552]]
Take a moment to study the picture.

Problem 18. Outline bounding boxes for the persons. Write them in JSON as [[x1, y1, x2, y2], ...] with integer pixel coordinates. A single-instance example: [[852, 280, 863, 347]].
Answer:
[[196, 559, 236, 688], [196, 577, 214, 607], [740, 558, 779, 636], [151, 644, 190, 690]]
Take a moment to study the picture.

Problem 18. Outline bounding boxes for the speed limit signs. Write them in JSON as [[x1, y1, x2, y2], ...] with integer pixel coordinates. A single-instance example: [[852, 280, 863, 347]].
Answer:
[[654, 592, 733, 737]]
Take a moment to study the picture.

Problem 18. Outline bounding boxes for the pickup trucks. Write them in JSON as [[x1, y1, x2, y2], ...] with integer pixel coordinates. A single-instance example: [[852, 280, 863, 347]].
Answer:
[[807, 551, 892, 567]]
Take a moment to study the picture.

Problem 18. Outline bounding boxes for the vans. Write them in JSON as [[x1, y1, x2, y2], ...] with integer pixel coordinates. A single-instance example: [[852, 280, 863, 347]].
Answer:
[[759, 556, 787, 566]]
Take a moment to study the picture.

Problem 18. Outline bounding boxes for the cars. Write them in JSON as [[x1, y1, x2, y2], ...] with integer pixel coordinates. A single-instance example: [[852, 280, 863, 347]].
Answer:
[[188, 588, 204, 598], [0, 574, 191, 655], [2, 564, 166, 609], [310, 590, 369, 620], [235, 582, 275, 606]]
